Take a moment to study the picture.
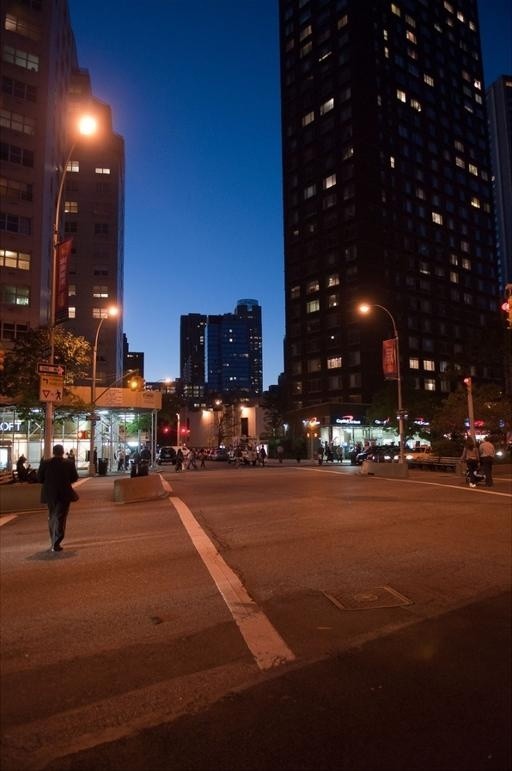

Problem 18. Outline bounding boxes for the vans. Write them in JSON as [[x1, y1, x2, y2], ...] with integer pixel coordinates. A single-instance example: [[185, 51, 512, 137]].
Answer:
[[166, 446, 190, 455]]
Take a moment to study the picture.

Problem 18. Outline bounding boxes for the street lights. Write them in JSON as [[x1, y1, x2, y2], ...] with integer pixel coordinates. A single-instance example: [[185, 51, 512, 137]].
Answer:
[[88, 306, 117, 476], [43, 114, 98, 459], [360, 304, 405, 465]]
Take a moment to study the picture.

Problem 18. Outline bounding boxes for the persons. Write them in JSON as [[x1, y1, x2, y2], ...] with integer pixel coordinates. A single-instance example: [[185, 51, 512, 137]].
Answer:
[[93, 448, 98, 473], [458, 435, 479, 489], [259, 446, 266, 466], [117, 447, 152, 472], [16, 456, 39, 484], [277, 445, 284, 464], [66, 449, 76, 462], [478, 437, 496, 488], [159, 447, 207, 470], [38, 445, 80, 554], [318, 442, 344, 465], [233, 446, 243, 466], [357, 442, 400, 453]]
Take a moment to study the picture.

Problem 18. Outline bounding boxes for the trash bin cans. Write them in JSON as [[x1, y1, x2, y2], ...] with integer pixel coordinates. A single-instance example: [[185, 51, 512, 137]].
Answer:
[[98, 458, 108, 475]]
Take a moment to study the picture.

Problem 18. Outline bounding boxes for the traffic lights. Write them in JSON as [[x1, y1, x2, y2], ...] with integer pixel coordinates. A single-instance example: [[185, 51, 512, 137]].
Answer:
[[464, 378, 470, 384], [502, 303, 510, 311], [130, 376, 145, 392]]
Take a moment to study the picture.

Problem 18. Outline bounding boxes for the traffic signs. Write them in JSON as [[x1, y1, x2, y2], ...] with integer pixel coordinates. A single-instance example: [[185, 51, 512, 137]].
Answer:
[[39, 363, 66, 376]]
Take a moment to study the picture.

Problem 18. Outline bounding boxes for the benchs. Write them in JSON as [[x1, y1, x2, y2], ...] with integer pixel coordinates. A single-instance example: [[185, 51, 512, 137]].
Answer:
[[433, 456, 464, 471], [1, 469, 19, 487]]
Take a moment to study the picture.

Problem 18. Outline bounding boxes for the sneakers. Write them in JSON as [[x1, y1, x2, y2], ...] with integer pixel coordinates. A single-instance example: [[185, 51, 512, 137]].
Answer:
[[52, 546, 63, 552], [470, 483, 476, 488]]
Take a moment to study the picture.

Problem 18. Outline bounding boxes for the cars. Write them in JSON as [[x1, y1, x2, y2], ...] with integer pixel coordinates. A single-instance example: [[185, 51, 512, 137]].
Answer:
[[356, 446, 432, 463], [204, 447, 258, 465]]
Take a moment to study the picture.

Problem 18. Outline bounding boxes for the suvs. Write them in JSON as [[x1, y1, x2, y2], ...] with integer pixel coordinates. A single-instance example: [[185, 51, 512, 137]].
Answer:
[[155, 448, 177, 465]]
